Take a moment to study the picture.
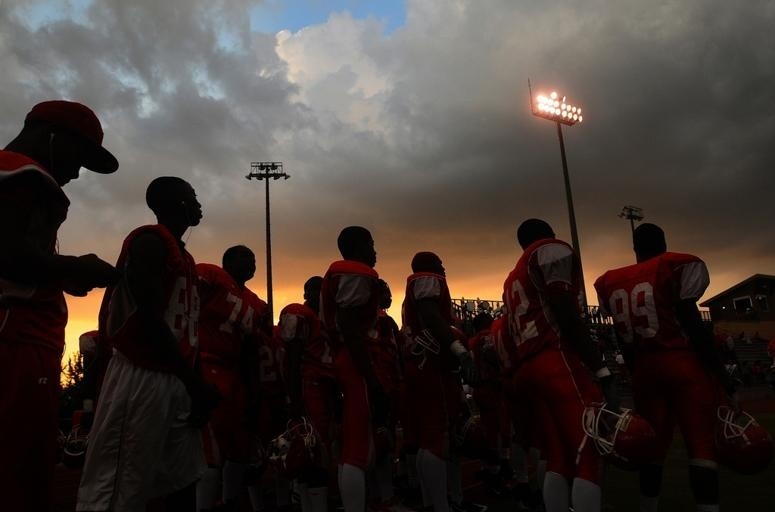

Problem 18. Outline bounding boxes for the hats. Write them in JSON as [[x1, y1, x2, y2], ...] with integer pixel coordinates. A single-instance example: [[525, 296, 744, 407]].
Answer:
[[26, 100, 119, 174]]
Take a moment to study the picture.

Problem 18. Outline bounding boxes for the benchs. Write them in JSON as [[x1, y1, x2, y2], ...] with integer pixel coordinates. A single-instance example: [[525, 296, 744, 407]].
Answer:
[[600, 324, 775, 396]]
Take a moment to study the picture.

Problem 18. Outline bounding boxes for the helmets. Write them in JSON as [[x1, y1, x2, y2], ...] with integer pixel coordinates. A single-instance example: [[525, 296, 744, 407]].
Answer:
[[267, 419, 326, 481], [414, 327, 466, 363], [714, 404, 774, 472], [581, 398, 650, 460], [59, 437, 88, 467]]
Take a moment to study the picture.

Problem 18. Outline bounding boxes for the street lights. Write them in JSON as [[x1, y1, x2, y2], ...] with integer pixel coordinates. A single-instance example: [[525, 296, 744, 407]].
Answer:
[[617, 204, 645, 234], [528, 78, 592, 318], [245, 161, 292, 329]]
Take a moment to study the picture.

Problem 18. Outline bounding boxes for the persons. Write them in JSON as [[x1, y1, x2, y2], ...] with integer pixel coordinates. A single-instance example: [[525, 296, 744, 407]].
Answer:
[[501, 216, 616, 510], [592, 223, 743, 511], [59, 244, 775, 511], [320, 225, 391, 510], [75, 175, 228, 512], [0, 99, 123, 511]]
